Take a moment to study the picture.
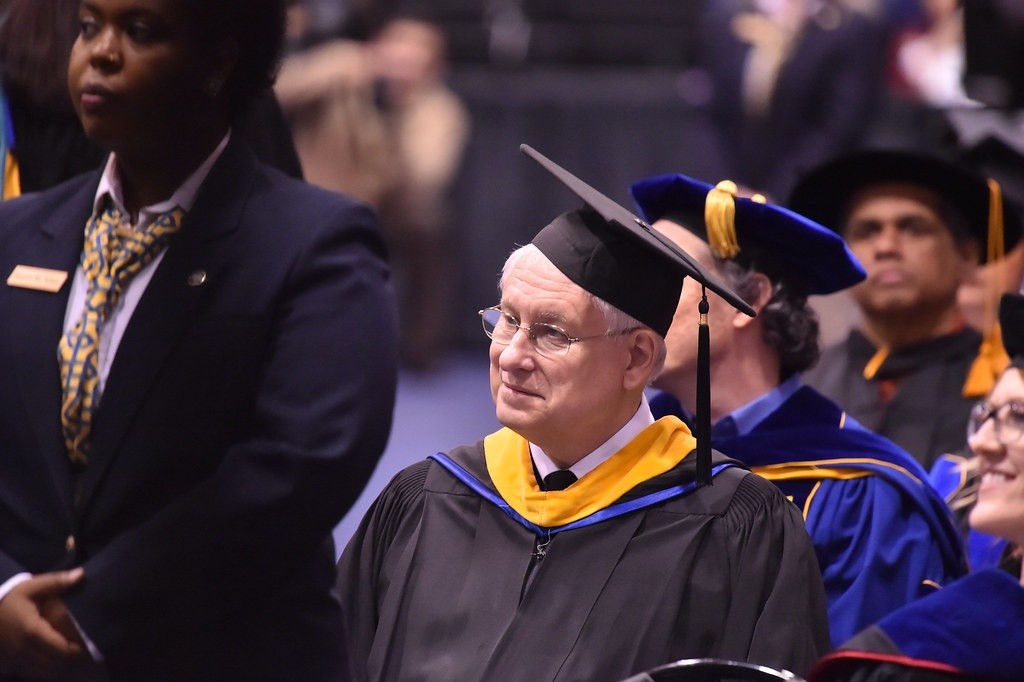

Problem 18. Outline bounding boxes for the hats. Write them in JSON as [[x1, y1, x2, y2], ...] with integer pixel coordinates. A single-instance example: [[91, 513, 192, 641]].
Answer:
[[788, 147, 1024, 400], [629, 172, 867, 296], [1000, 293, 1022, 369], [518, 144, 757, 488]]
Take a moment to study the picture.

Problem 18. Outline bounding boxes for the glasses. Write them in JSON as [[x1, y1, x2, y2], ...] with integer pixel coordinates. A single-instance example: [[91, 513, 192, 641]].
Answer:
[[478, 306, 647, 362], [966, 401, 1024, 448]]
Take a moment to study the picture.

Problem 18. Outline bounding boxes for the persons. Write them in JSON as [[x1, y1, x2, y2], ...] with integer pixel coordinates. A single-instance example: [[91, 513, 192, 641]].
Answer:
[[789, 145, 1005, 474], [276, 18, 471, 362], [0, 0, 403, 682], [709, 0, 968, 162], [627, 173, 968, 646], [811, 288, 1024, 682], [334, 144, 832, 682]]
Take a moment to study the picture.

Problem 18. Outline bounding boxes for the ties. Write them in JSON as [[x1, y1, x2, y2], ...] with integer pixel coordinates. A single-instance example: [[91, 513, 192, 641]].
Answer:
[[59, 200, 183, 464], [544, 469, 577, 491]]
